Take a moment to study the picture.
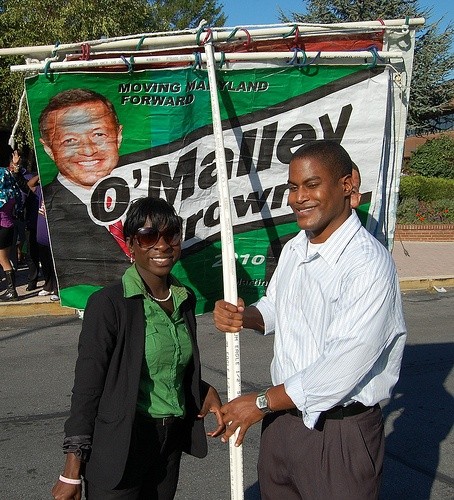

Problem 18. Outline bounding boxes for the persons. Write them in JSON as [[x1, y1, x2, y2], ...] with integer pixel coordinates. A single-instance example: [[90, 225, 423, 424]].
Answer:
[[263, 161, 388, 297], [39, 89, 136, 292], [0, 144, 60, 302], [51, 196, 226, 500], [213, 139, 408, 500]]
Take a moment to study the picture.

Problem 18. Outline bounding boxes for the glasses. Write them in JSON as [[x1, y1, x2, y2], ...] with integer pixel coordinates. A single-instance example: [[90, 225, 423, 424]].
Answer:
[[133, 225, 183, 249]]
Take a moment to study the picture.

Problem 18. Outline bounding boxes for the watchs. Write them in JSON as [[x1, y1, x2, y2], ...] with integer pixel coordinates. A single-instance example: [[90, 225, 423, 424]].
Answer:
[[255, 388, 277, 415]]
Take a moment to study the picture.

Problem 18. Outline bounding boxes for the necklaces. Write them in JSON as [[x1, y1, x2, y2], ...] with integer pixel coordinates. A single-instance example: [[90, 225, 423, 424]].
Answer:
[[147, 289, 172, 302]]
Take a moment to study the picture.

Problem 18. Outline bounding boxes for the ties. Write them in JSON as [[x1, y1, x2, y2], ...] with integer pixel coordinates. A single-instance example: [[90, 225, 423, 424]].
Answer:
[[106, 197, 131, 261]]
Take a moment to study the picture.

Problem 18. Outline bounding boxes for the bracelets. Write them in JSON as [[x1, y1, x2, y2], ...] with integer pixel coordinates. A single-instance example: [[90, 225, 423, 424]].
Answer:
[[59, 475, 82, 484]]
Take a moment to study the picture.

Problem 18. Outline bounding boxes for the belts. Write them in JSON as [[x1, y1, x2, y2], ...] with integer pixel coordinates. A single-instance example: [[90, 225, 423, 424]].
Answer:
[[286, 401, 373, 420]]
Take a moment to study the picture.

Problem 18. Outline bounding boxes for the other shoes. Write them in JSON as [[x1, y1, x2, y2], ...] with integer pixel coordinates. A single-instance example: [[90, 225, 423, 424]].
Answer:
[[37, 289, 55, 296], [50, 294, 60, 301], [26, 286, 38, 291]]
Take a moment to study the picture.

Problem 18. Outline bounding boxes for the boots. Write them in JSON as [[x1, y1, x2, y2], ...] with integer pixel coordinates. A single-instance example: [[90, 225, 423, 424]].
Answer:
[[0, 271, 19, 301]]
